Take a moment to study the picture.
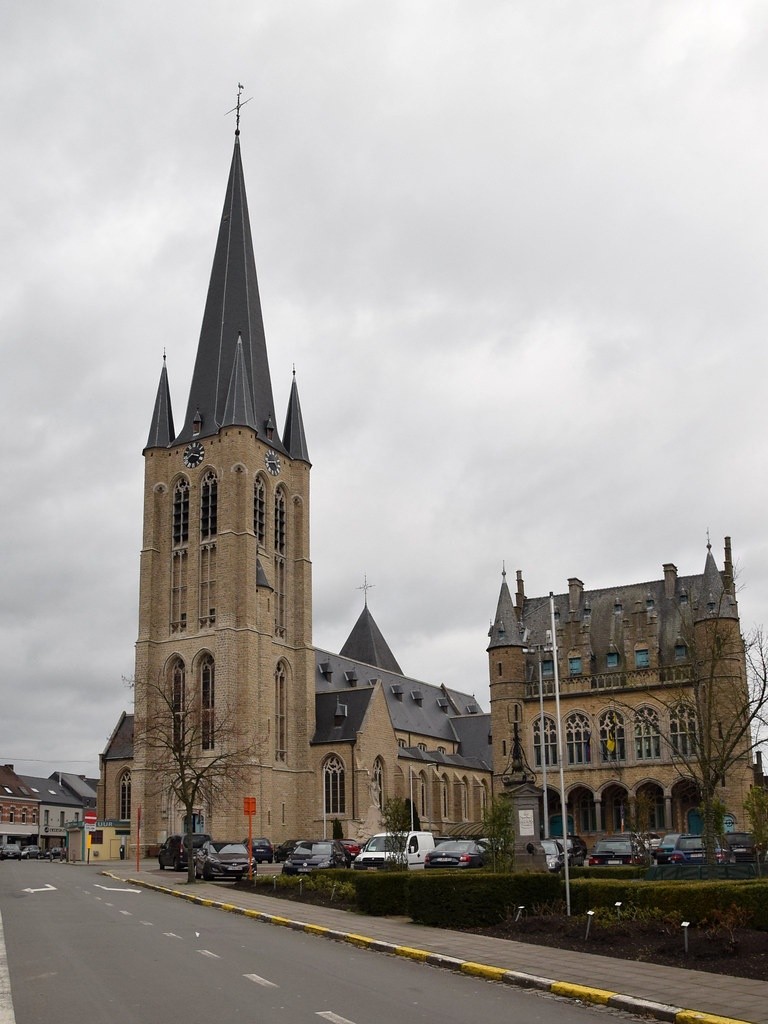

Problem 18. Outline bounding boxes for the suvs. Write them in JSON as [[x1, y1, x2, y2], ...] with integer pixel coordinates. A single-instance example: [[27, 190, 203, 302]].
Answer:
[[724, 830, 766, 863], [670, 833, 726, 864]]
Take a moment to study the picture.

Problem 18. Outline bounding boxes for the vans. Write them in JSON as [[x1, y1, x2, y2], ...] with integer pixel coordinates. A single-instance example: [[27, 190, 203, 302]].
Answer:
[[354, 831, 436, 872], [157, 833, 213, 872]]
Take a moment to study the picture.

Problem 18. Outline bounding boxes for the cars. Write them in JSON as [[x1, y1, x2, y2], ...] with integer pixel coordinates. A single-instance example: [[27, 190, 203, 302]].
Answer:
[[1, 844, 21, 860], [657, 834, 687, 864], [424, 838, 496, 871], [281, 840, 352, 878], [20, 845, 43, 859], [338, 838, 361, 859], [625, 831, 662, 853], [532, 834, 588, 872], [590, 838, 656, 866], [44, 846, 66, 858], [274, 839, 313, 864], [192, 840, 258, 881], [242, 836, 275, 863]]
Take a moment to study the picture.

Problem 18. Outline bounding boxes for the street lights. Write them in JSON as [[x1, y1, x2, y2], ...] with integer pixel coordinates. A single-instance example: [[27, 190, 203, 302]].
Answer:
[[520, 645, 559, 839]]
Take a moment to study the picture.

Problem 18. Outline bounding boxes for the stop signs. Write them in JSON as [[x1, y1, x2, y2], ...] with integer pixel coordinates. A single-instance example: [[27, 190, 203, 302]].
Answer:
[[84, 811, 98, 824]]
